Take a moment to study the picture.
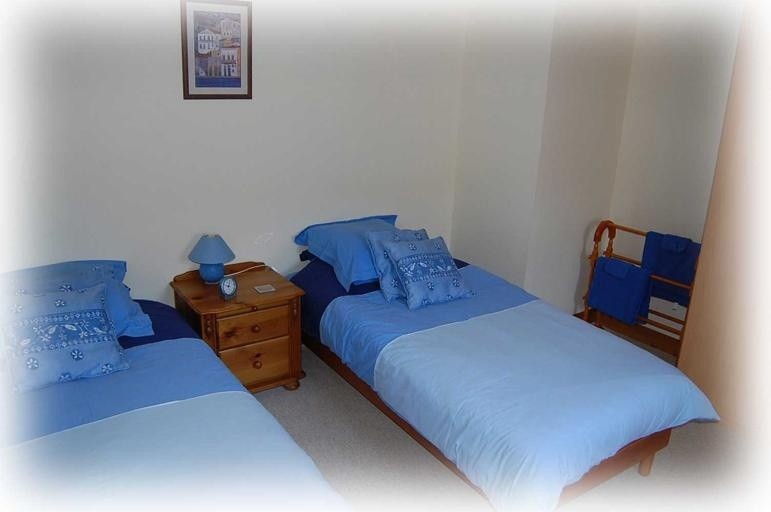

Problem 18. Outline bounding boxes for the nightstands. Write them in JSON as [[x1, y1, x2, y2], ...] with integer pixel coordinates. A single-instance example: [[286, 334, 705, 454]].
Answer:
[[169, 260, 306, 395]]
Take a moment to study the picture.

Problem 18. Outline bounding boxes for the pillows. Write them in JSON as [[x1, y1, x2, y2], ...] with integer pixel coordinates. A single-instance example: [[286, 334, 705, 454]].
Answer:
[[380, 236, 471, 311], [0, 260, 154, 339], [364, 228, 430, 304], [2, 284, 129, 400], [294, 215, 398, 293]]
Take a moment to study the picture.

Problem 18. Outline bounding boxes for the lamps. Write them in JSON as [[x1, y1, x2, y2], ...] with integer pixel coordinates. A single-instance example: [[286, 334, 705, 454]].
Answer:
[[187, 232, 237, 285]]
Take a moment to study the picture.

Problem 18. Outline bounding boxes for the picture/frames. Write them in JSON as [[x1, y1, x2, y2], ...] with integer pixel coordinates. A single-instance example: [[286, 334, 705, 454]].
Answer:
[[179, 0, 252, 100]]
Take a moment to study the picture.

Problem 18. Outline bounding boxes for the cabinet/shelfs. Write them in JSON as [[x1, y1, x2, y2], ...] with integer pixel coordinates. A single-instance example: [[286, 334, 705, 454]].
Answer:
[[582, 220, 699, 369]]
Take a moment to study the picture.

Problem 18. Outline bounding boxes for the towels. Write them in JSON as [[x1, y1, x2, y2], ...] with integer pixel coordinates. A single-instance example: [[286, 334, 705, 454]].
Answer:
[[644, 230, 702, 307], [587, 256, 648, 325]]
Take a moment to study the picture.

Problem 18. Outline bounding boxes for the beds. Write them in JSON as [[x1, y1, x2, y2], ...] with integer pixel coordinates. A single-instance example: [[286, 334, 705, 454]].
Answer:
[[289, 254, 721, 512], [0, 300, 338, 512]]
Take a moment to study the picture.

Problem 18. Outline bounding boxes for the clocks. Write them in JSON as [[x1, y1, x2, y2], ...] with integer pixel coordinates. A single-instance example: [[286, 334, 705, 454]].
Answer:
[[219, 276, 238, 302]]
